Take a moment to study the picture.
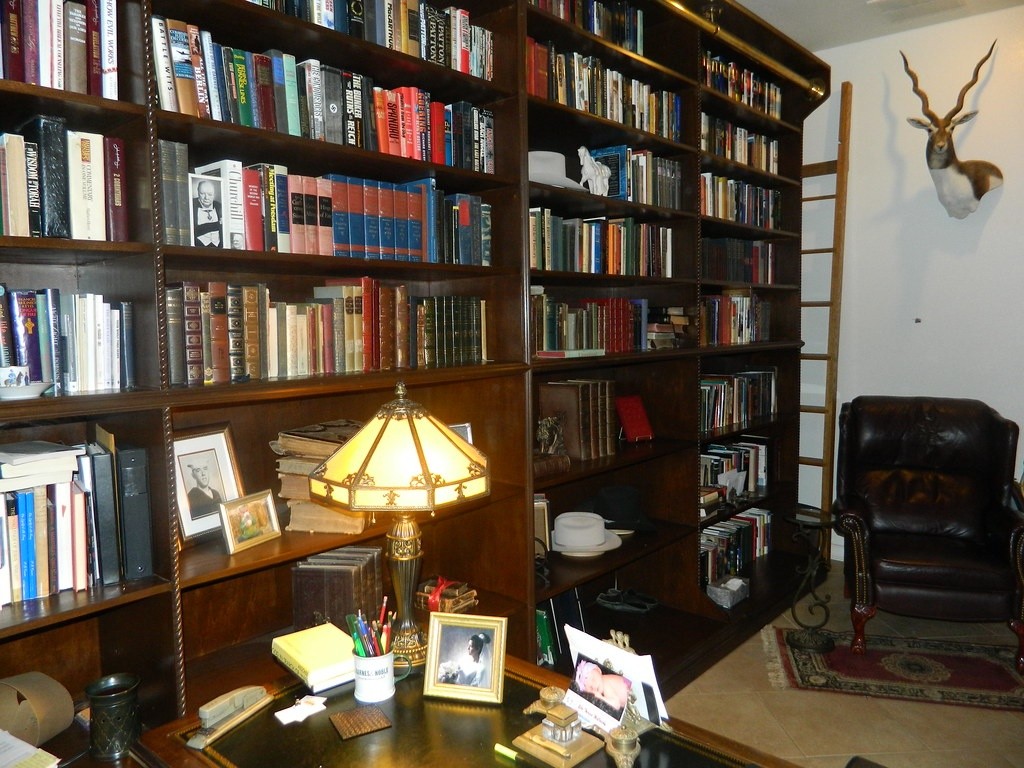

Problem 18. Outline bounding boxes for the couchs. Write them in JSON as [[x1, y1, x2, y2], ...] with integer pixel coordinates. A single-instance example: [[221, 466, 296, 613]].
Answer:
[[830, 394, 1024, 671]]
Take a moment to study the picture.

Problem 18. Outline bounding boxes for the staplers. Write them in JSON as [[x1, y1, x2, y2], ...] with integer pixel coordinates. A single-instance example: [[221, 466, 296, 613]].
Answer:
[[186, 685, 275, 751]]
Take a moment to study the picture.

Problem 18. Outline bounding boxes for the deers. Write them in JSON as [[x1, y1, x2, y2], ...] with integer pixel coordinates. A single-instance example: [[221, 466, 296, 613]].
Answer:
[[899, 39, 1004, 219]]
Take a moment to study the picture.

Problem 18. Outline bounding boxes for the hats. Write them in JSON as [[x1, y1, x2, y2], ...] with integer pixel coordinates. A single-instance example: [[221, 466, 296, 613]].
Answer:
[[551, 512, 622, 552], [187, 459, 207, 470]]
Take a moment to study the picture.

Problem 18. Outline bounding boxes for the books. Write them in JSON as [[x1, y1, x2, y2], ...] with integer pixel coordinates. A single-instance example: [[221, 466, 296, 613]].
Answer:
[[700, 363, 778, 432], [700, 173, 784, 231], [700, 507, 771, 590], [534, 493, 549, 556], [272, 546, 384, 694], [528, 0, 644, 57], [1, 0, 497, 614], [702, 238, 780, 285], [702, 50, 782, 119], [699, 288, 771, 347], [536, 608, 556, 666], [701, 434, 782, 522], [1, 729, 62, 768], [527, 34, 689, 480], [701, 112, 779, 175]]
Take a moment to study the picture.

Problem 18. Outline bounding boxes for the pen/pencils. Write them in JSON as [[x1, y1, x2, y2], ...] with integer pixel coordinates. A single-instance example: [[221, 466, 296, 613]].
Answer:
[[493, 743, 550, 768], [345, 596, 397, 658]]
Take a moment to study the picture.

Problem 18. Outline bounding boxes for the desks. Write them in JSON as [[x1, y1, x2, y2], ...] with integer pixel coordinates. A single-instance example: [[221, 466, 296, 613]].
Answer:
[[75, 623, 811, 767]]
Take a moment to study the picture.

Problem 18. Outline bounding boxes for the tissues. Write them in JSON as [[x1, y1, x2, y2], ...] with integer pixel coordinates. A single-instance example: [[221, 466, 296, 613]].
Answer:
[[706, 572, 751, 609]]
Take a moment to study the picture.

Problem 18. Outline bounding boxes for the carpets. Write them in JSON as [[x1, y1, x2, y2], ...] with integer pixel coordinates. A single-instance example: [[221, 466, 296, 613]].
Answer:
[[759, 622, 1024, 712]]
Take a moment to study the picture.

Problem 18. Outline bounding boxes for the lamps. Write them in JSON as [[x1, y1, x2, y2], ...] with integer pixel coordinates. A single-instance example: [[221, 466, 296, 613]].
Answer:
[[309, 382, 490, 669]]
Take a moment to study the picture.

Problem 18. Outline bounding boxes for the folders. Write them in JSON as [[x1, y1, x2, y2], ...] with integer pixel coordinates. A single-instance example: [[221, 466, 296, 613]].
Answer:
[[85, 440, 122, 587], [116, 442, 154, 582]]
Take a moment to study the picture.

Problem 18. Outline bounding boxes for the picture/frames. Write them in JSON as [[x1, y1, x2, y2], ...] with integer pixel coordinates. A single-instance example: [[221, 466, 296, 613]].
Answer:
[[214, 489, 282, 555], [422, 610, 508, 704], [173, 420, 247, 550]]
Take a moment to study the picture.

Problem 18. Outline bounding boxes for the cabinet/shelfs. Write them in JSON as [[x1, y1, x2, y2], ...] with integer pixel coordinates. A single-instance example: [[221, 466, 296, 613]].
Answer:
[[0, 0, 838, 768]]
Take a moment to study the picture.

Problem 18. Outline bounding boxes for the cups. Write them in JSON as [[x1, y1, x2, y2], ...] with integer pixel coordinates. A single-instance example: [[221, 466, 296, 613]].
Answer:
[[351, 647, 413, 704]]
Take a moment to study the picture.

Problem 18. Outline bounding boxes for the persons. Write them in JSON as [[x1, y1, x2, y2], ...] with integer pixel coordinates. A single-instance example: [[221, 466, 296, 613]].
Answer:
[[237, 504, 263, 542], [437, 632, 492, 687], [187, 459, 223, 518], [192, 179, 223, 247], [575, 661, 630, 710]]
